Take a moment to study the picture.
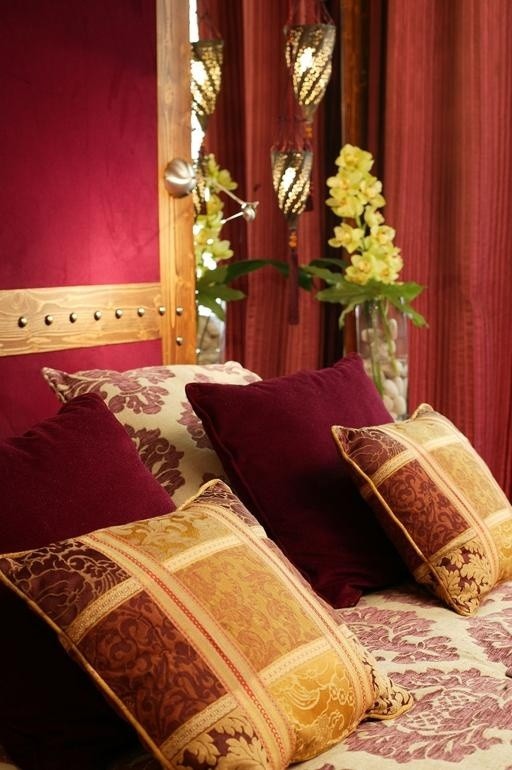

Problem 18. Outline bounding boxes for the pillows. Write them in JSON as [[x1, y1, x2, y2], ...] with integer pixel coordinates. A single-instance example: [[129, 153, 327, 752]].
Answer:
[[2, 454, 413, 770], [0, 395, 178, 770], [327, 399, 512, 621], [41, 353, 265, 508], [184, 348, 396, 609]]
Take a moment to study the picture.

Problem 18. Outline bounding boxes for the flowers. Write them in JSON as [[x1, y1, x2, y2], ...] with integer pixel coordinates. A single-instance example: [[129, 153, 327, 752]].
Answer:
[[297, 141, 429, 403], [189, 155, 292, 363]]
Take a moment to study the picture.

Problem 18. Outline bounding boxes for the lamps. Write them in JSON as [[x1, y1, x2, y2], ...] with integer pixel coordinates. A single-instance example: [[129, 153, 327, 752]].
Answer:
[[268, 0, 342, 247], [187, 10, 228, 134]]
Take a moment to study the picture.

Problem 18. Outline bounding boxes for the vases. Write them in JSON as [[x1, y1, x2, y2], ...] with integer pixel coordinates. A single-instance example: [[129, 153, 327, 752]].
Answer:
[[196, 297, 228, 365], [351, 301, 411, 421]]
